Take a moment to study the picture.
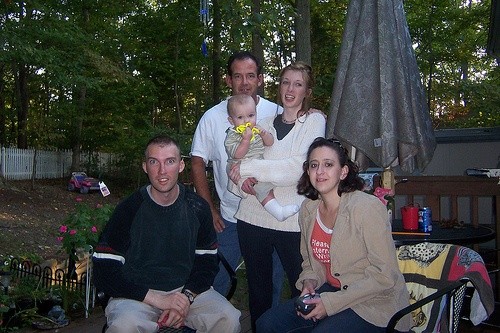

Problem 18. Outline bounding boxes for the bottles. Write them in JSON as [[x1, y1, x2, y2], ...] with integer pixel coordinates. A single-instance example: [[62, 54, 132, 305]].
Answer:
[[418, 206, 433, 233]]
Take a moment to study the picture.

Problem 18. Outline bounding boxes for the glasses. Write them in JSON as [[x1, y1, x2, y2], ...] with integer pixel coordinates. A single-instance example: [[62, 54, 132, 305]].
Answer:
[[313, 137, 346, 153]]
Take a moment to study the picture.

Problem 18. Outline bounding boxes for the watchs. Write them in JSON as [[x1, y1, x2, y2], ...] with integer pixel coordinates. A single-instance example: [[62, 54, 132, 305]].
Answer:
[[181, 290, 194, 305]]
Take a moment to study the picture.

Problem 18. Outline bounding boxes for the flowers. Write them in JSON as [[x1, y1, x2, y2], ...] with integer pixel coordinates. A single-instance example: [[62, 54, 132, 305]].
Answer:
[[374, 187, 395, 205]]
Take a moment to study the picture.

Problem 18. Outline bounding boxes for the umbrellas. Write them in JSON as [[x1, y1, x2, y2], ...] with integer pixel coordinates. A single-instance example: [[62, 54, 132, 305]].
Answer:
[[325, 0, 437, 197]]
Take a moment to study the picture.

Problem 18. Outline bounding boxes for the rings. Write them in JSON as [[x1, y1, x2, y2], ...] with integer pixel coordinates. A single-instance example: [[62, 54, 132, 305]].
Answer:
[[248, 183, 255, 189]]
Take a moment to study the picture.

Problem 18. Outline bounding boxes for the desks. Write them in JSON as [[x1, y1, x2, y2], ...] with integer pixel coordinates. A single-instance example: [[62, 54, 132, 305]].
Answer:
[[392, 219, 495, 243]]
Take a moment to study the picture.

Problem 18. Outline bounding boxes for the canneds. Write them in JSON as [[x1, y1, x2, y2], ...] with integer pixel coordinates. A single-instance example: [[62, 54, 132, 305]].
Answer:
[[418, 206, 432, 233]]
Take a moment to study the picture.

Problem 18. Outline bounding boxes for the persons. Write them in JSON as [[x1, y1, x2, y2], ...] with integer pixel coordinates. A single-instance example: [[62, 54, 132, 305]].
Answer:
[[224, 94, 300, 221], [187, 51, 286, 308], [92, 135, 241, 333], [255, 137, 412, 333], [226, 63, 327, 333]]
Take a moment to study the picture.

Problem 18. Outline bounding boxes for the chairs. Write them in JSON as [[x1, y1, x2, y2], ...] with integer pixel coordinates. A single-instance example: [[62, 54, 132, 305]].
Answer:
[[103, 253, 238, 332], [387, 243, 469, 333]]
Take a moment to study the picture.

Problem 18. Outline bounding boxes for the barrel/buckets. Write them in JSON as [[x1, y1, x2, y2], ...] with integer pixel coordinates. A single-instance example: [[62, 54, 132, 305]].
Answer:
[[400, 202, 420, 230]]
